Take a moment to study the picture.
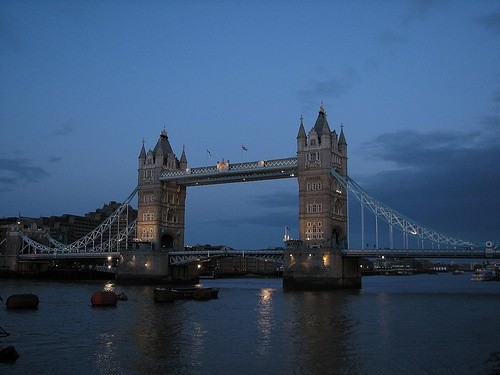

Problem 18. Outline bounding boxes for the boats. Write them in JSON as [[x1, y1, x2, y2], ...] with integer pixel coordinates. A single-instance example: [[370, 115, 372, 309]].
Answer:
[[168, 284, 221, 300]]
